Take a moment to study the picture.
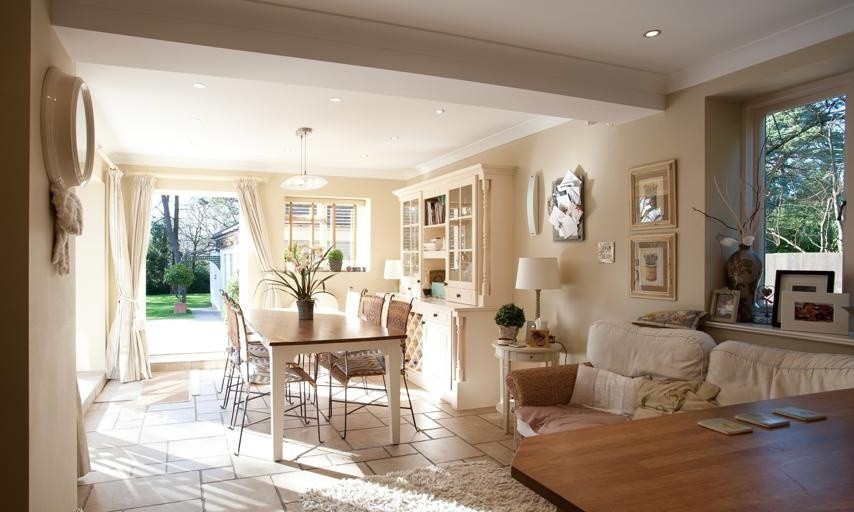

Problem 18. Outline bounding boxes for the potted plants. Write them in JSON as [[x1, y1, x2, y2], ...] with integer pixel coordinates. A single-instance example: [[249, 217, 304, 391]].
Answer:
[[163, 264, 196, 314], [494, 303, 526, 346], [328, 250, 344, 272]]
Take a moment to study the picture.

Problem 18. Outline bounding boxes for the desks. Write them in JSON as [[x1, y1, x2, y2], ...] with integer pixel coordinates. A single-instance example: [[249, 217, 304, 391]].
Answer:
[[510, 388, 854, 512]]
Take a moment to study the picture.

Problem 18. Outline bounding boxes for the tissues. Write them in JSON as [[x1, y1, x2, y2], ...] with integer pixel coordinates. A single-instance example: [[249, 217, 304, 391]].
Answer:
[[529, 317, 550, 348]]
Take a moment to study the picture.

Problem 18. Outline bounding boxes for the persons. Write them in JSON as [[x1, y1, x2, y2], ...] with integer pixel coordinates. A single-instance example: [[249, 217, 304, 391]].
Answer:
[[717, 297, 734, 319]]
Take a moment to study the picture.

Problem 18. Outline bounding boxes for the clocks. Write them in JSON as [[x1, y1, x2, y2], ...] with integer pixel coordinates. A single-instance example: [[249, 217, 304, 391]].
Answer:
[[41, 67, 95, 193]]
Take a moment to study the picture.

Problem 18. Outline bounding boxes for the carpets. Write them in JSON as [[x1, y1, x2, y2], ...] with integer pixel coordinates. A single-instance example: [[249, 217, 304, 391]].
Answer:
[[300, 458, 558, 511]]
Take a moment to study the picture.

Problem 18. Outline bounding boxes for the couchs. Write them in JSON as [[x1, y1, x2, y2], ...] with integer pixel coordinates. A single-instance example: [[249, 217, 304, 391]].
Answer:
[[504, 318, 854, 455]]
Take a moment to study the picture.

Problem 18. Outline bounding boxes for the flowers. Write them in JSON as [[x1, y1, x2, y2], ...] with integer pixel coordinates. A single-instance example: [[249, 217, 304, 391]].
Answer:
[[252, 244, 338, 307]]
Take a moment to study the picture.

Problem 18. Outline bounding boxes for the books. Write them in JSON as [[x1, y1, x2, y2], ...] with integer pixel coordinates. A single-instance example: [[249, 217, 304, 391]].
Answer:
[[734, 410, 790, 429], [427, 201, 445, 226], [773, 406, 828, 423], [697, 417, 754, 436]]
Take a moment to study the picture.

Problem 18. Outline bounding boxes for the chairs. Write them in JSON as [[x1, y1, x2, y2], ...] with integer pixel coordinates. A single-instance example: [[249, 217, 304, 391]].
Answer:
[[218, 289, 421, 457]]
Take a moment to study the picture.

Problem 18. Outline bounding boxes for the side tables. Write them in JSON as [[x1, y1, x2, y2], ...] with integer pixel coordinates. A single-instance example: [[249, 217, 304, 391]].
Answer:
[[492, 340, 564, 414]]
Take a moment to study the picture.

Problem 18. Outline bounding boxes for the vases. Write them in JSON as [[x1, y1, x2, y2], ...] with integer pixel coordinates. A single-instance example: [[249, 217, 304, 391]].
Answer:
[[727, 241, 763, 322]]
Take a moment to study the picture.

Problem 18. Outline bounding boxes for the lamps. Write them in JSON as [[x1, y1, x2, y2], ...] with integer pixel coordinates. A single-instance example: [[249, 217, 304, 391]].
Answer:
[[384, 259, 401, 293], [514, 257, 562, 321], [280, 128, 328, 190]]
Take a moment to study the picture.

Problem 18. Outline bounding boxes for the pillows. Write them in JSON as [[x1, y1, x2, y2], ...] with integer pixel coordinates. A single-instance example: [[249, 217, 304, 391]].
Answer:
[[569, 310, 854, 420]]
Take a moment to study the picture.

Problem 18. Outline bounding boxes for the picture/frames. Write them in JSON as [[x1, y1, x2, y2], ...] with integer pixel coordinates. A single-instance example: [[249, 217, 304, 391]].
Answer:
[[628, 233, 678, 301], [628, 160, 680, 232], [709, 286, 741, 323], [772, 270, 850, 335]]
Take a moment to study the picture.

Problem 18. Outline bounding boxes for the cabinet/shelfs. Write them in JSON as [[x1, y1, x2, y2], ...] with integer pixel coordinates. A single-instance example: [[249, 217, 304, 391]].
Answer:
[[392, 163, 518, 412]]
